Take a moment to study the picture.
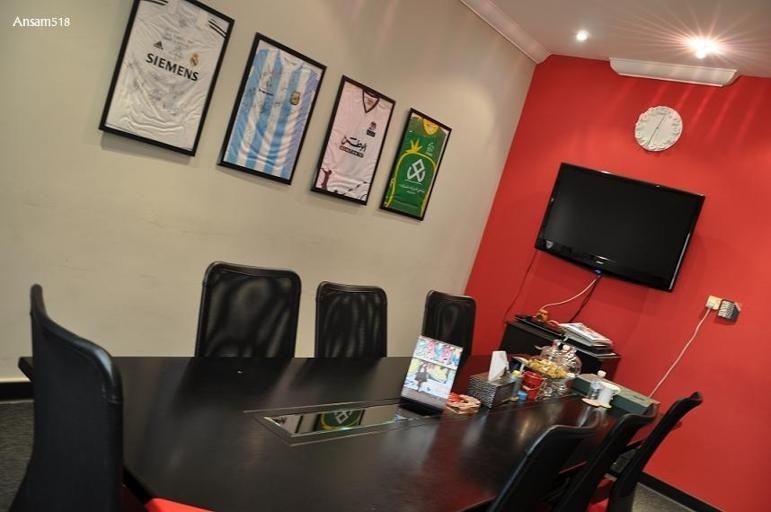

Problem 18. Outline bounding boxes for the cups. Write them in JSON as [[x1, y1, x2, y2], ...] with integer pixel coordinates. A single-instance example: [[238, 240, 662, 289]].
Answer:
[[597, 382, 622, 406]]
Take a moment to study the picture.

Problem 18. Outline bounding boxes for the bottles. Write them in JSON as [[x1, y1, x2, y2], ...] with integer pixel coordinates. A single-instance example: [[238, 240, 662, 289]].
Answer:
[[539, 339, 564, 364], [587, 370, 606, 401]]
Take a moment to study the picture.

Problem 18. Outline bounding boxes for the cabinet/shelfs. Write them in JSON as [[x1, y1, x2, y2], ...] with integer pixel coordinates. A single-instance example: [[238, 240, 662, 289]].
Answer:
[[496, 320, 620, 386]]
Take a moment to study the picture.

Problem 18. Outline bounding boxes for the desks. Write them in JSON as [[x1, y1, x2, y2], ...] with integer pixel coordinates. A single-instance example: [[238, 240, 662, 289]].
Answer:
[[18, 353, 659, 512]]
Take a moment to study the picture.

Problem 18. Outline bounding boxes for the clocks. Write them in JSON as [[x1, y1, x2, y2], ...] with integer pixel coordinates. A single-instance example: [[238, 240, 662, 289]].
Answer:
[[633, 106, 682, 152]]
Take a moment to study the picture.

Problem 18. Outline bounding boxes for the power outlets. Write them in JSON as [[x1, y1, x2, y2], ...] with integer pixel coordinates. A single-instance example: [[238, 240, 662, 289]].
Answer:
[[707, 295, 722, 312]]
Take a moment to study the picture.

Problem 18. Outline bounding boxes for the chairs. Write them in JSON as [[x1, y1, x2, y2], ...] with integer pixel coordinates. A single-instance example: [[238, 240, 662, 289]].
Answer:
[[480, 411, 603, 510], [420, 290, 476, 354], [193, 262, 301, 358], [313, 279, 388, 357], [556, 404, 659, 512], [7, 282, 129, 512], [607, 392, 703, 510]]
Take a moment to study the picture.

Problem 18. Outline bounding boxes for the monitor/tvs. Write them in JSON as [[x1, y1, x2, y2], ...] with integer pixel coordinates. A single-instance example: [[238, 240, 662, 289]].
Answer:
[[534, 161, 707, 293]]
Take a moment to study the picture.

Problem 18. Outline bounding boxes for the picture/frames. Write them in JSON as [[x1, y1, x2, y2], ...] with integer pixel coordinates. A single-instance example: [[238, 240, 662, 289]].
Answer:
[[216, 32, 327, 186], [309, 74, 397, 204], [379, 107, 453, 222], [98, 0, 236, 156]]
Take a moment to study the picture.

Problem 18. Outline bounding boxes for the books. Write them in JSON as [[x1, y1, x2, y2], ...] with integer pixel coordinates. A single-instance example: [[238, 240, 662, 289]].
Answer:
[[558, 322, 613, 347]]
